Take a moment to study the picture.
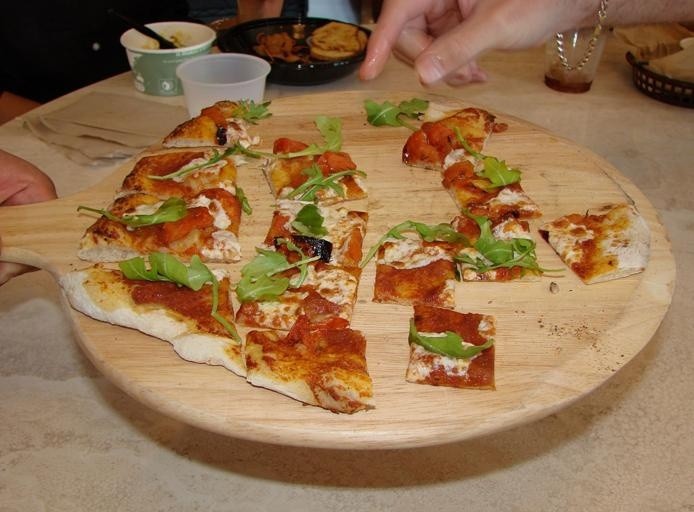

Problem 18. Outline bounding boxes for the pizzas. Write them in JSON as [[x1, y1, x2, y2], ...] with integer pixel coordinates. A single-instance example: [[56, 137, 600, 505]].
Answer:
[[62, 99, 651, 414]]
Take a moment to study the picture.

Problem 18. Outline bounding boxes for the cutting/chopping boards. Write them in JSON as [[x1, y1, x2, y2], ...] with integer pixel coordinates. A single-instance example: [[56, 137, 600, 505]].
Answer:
[[0, 91, 678, 488]]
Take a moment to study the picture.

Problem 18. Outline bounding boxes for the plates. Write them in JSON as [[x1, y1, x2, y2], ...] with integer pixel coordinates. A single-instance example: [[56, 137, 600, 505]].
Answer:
[[213, 17, 373, 85]]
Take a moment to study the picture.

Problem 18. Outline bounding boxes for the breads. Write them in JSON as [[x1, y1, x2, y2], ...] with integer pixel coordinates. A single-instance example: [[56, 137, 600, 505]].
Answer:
[[613, 22, 694, 63], [649, 38, 694, 84]]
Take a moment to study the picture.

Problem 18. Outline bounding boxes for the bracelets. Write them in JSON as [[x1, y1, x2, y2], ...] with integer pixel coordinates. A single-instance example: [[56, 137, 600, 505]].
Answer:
[[556, 0, 608, 72]]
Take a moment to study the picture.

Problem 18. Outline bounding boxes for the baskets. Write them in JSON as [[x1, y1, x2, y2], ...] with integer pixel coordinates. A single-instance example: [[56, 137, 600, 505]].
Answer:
[[625, 51, 694, 109]]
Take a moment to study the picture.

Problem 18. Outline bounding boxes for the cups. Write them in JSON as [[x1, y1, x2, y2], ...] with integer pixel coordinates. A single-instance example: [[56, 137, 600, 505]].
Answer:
[[543, 24, 609, 92], [119, 18, 272, 120]]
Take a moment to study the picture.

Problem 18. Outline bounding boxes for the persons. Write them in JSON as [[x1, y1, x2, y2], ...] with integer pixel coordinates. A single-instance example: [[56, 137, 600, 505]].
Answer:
[[147, 1, 362, 30], [361, 0, 694, 87]]
